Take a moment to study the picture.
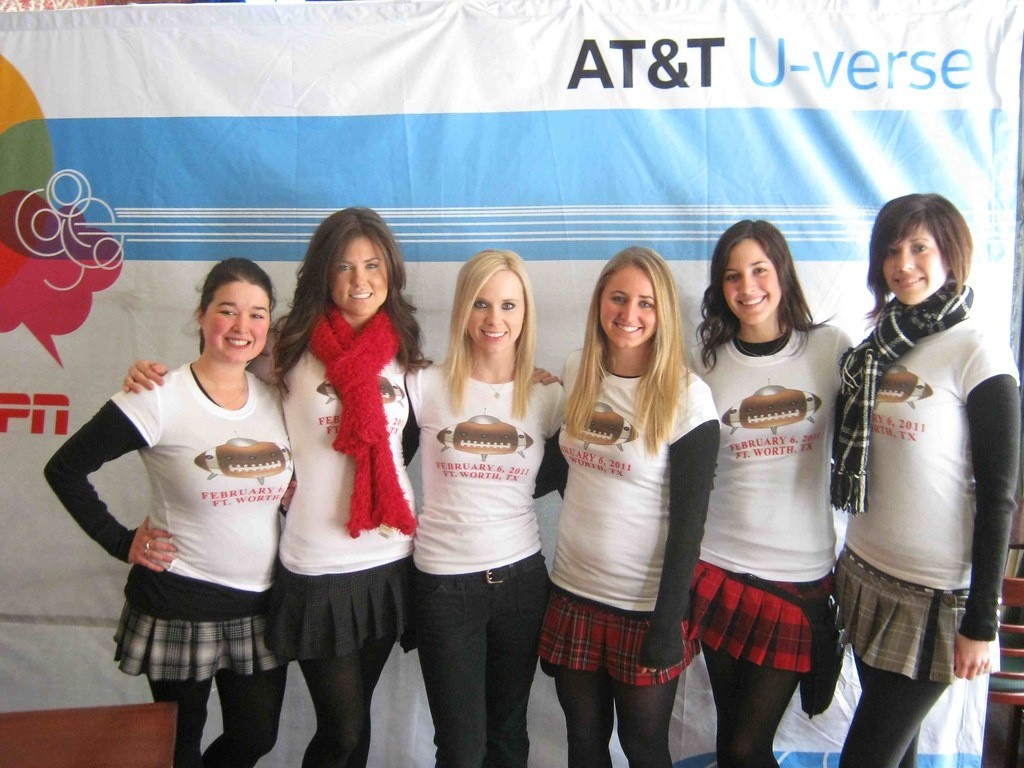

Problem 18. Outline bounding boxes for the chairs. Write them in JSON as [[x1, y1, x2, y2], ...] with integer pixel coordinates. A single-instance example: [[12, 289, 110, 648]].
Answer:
[[983, 576, 1024, 768]]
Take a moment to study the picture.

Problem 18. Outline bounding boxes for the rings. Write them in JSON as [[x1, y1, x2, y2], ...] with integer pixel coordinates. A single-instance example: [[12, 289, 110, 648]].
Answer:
[[144, 549, 150, 558], [146, 539, 152, 549]]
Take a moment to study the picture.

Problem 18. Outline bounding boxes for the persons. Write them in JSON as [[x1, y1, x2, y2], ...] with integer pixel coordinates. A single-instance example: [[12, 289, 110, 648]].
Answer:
[[281, 250, 569, 767], [532, 246, 720, 768], [246, 328, 283, 384], [686, 220, 854, 768], [42, 258, 296, 768], [124, 207, 563, 768], [835, 194, 1022, 768]]
